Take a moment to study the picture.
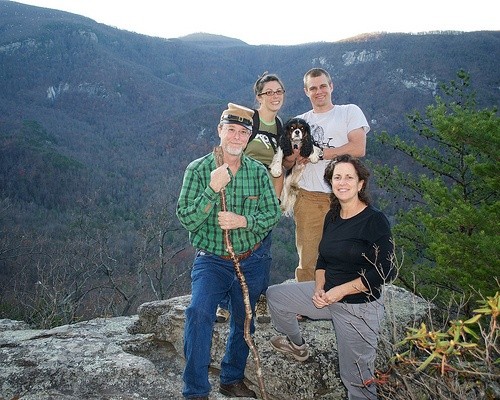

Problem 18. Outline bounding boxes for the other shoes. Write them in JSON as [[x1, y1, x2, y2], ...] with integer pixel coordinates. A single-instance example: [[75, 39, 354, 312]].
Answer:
[[297, 314, 308, 321]]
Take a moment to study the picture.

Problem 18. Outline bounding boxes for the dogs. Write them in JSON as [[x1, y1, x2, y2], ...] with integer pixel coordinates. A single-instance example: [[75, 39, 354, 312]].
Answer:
[[268, 118, 321, 218]]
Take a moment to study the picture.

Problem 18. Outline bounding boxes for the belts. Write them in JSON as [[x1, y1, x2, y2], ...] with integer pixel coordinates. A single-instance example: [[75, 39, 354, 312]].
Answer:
[[220, 243, 260, 261]]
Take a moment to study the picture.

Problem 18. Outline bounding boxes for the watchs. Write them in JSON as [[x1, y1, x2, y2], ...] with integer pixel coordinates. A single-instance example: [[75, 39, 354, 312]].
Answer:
[[319, 148, 324, 160]]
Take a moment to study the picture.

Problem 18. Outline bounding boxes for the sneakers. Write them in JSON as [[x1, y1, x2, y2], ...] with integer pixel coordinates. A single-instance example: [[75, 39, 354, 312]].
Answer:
[[219, 381, 257, 399], [269, 335, 309, 361]]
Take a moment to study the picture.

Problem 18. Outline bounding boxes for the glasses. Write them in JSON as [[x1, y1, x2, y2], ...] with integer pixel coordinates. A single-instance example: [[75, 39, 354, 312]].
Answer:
[[258, 89, 285, 96]]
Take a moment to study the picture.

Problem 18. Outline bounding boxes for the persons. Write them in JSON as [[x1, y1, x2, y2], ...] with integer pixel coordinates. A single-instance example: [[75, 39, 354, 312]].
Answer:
[[177, 103, 280, 400], [265, 155, 394, 400], [215, 73, 291, 323], [284, 69, 371, 324]]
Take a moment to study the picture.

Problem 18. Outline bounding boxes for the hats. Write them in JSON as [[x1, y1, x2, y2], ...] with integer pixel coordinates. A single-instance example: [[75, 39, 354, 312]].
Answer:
[[220, 103, 255, 130]]
